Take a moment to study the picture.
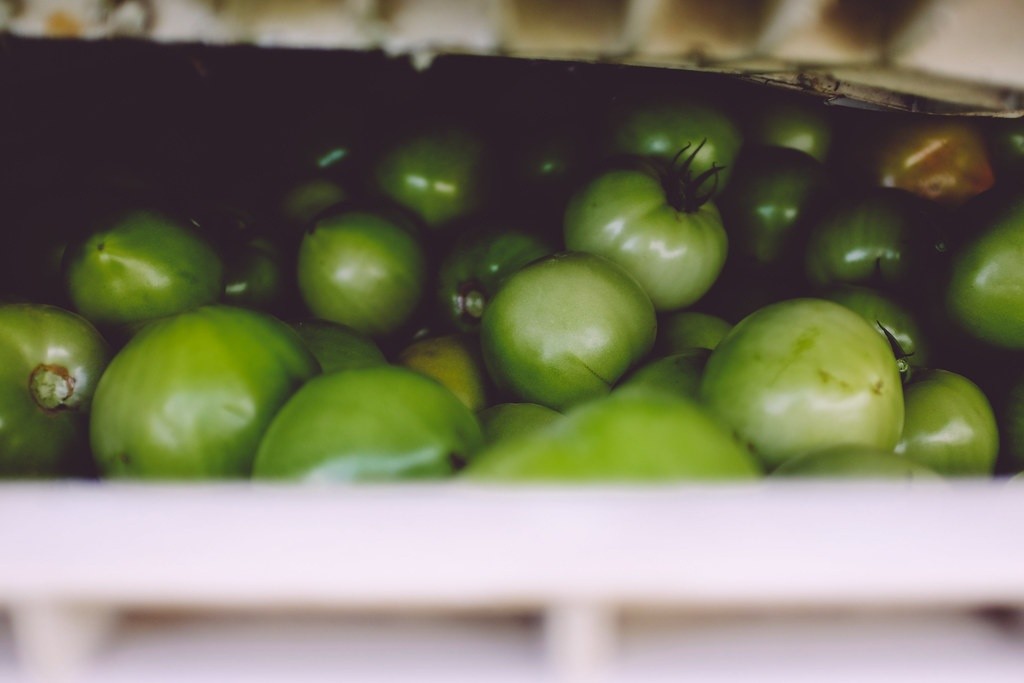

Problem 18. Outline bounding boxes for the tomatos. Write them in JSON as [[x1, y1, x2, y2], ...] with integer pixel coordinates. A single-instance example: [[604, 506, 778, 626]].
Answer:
[[0, 45, 1024, 485]]
